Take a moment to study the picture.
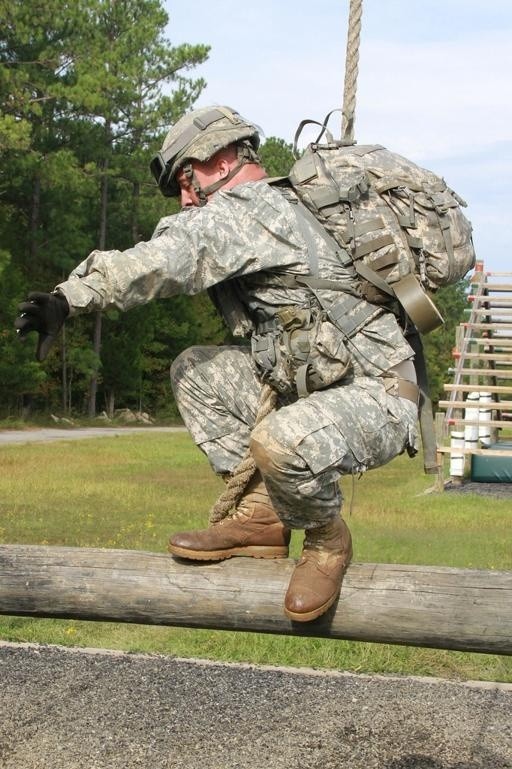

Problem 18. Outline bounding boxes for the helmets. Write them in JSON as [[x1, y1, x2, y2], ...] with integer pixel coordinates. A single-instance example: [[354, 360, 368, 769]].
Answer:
[[162, 105, 261, 195]]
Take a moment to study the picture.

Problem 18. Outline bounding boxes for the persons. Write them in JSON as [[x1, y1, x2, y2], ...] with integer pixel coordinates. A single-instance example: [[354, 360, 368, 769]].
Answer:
[[14, 104, 421, 623]]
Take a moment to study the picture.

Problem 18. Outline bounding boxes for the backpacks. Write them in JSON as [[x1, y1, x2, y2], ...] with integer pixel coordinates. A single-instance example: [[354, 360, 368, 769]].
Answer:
[[241, 110, 476, 305]]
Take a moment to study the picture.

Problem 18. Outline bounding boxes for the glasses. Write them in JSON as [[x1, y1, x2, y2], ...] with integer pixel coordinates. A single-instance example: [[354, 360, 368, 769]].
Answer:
[[150, 107, 238, 185]]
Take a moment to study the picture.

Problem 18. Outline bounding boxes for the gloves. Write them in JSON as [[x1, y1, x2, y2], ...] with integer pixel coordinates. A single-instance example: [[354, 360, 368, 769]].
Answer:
[[14, 292, 69, 362]]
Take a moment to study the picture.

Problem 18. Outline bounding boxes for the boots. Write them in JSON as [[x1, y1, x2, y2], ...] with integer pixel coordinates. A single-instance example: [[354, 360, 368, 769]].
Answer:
[[167, 500, 291, 562], [284, 516, 352, 622]]
